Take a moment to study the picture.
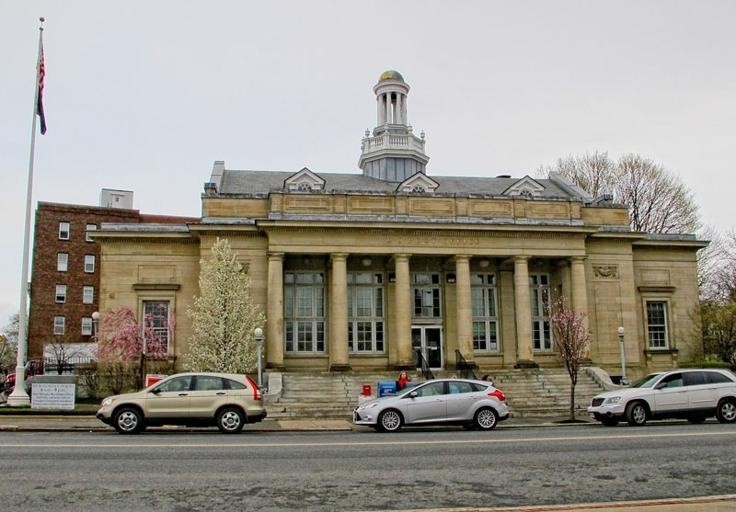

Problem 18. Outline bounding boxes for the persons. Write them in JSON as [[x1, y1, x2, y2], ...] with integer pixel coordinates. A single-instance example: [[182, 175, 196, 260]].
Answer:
[[395, 369, 412, 392], [448, 376, 459, 393]]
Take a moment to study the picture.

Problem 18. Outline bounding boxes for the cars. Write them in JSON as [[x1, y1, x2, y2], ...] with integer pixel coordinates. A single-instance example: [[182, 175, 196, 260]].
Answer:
[[588, 368, 736, 426], [96, 372, 267, 434], [353, 379, 509, 431]]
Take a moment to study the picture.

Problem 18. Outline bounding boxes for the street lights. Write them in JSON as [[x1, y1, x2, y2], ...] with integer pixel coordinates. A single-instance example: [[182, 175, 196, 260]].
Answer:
[[92, 312, 99, 343], [255, 328, 265, 385], [617, 327, 627, 384]]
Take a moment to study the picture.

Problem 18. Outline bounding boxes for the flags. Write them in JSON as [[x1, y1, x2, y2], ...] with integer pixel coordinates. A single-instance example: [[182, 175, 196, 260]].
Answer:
[[33, 38, 47, 135]]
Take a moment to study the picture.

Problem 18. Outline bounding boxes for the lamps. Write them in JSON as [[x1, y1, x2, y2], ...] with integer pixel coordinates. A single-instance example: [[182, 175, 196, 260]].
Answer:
[[361, 256, 373, 267], [479, 257, 490, 268], [447, 273, 456, 283], [388, 272, 396, 282]]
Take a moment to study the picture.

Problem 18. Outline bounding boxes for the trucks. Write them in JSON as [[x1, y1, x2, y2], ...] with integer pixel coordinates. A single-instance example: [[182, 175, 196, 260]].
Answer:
[[4, 343, 98, 389]]
